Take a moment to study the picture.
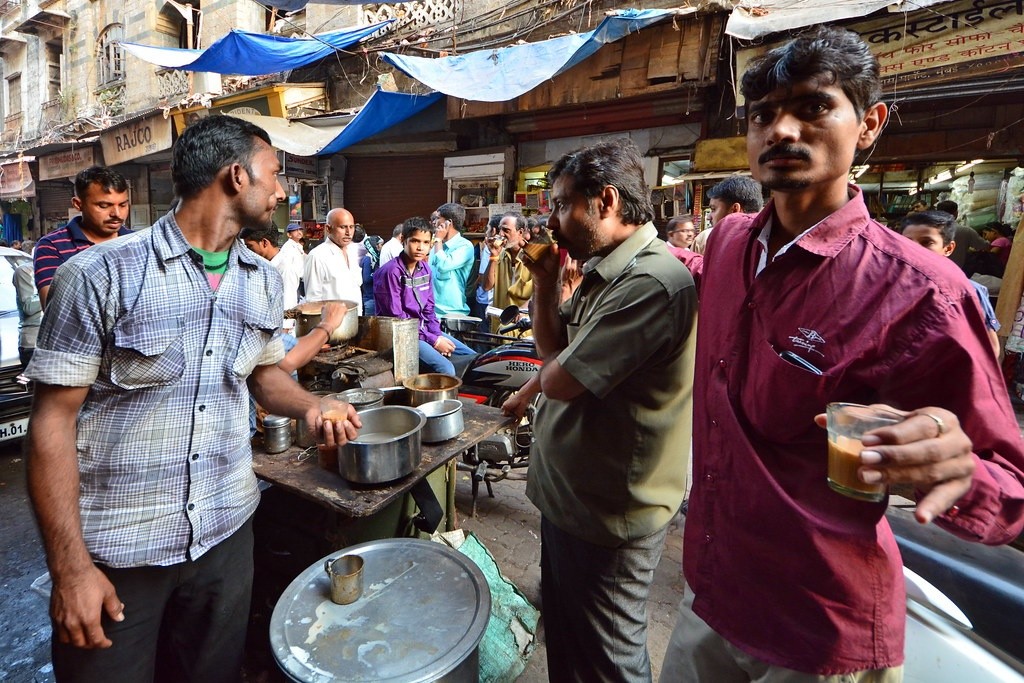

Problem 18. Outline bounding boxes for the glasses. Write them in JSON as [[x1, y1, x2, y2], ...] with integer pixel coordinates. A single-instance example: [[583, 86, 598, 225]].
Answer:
[[672, 229, 696, 234]]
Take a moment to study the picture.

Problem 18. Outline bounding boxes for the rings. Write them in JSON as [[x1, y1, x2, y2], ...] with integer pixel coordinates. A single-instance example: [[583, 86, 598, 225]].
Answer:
[[921, 412, 945, 438]]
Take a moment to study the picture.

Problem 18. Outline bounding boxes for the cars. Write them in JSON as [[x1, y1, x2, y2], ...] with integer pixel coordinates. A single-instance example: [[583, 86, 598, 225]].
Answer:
[[0, 245, 51, 450]]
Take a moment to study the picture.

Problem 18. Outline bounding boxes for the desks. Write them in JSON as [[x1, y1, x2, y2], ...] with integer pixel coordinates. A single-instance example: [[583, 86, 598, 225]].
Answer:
[[250, 399, 513, 538]]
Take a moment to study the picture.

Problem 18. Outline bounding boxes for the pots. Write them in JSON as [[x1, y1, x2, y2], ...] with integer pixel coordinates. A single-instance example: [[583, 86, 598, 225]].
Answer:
[[284, 300, 360, 345]]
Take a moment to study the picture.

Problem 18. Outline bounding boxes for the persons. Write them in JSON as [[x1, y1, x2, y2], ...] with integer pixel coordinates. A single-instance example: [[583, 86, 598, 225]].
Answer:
[[897, 200, 1012, 294], [241, 202, 582, 389], [668, 23, 1024, 682], [21, 114, 364, 683], [689, 175, 763, 255], [0, 237, 35, 254], [33, 166, 138, 316], [497, 136, 697, 683], [668, 216, 694, 246], [12, 261, 45, 370]]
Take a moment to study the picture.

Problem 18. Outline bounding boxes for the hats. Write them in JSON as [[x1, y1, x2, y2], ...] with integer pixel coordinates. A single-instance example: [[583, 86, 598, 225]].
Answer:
[[286, 223, 304, 231]]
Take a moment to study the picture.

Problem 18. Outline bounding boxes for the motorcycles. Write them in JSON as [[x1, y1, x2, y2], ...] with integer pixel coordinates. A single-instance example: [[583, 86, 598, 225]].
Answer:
[[419, 304, 548, 520]]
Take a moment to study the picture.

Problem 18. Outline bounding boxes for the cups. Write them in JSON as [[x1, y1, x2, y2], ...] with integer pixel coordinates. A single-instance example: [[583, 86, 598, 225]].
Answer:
[[826, 403, 906, 502], [493, 235, 505, 248], [320, 393, 349, 428], [324, 554, 365, 604], [521, 226, 558, 263]]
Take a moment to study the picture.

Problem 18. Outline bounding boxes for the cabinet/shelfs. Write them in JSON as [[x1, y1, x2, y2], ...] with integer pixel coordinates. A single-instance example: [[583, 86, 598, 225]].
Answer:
[[448, 175, 506, 236]]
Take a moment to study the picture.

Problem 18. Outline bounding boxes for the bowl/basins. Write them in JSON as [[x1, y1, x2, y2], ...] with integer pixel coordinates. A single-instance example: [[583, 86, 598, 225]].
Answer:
[[296, 419, 315, 448], [403, 373, 462, 406], [417, 399, 465, 443]]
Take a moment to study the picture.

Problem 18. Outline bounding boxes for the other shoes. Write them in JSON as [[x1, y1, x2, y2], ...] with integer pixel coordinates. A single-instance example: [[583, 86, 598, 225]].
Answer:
[[680, 499, 689, 515]]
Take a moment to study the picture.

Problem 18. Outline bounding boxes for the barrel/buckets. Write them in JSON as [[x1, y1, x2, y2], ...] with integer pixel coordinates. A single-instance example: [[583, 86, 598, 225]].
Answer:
[[338, 405, 427, 484], [269, 537, 493, 683]]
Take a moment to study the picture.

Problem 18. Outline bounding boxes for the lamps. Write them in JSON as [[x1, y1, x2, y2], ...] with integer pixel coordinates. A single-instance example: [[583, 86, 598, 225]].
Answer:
[[851, 163, 869, 185], [929, 158, 984, 184]]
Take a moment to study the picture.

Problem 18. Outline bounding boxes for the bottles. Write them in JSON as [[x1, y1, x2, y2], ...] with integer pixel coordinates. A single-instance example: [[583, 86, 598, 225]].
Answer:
[[262, 414, 292, 454]]
